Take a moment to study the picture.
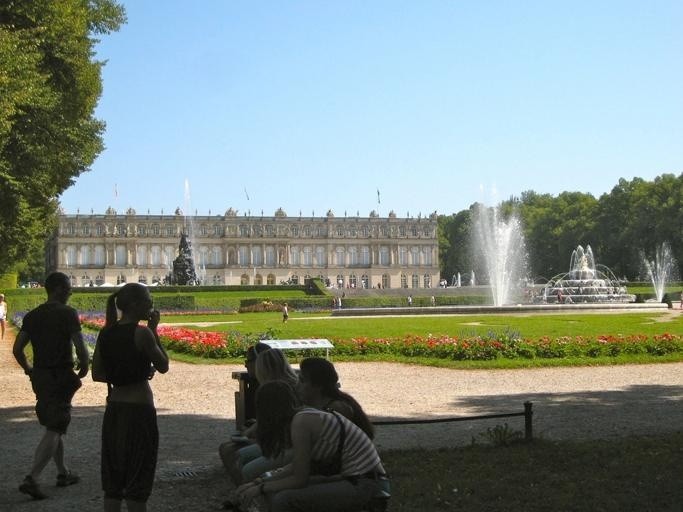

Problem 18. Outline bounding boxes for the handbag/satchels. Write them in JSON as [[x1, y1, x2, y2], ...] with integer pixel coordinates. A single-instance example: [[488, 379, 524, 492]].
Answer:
[[311, 454, 342, 475]]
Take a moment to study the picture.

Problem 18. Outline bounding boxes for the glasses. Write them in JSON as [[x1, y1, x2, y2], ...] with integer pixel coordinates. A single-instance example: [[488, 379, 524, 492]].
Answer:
[[245, 359, 253, 364]]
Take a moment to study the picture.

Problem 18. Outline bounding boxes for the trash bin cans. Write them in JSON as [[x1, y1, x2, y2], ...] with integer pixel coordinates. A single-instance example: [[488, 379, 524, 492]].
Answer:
[[231, 338, 340, 436]]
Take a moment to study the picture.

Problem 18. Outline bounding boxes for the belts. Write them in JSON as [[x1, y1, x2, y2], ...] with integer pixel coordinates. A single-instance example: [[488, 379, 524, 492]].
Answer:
[[361, 471, 388, 481]]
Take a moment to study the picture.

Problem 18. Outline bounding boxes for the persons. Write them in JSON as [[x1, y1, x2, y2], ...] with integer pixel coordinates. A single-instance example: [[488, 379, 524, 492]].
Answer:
[[431, 294, 436, 306], [680, 290, 683, 309], [428, 278, 432, 289], [557, 291, 563, 303], [13, 271, 89, 498], [440, 279, 448, 288], [324, 279, 366, 290], [93, 283, 170, 511], [282, 302, 288, 324], [16, 279, 41, 288], [219, 342, 392, 510], [0, 294, 8, 339], [378, 282, 385, 289], [408, 294, 412, 307], [331, 292, 346, 310]]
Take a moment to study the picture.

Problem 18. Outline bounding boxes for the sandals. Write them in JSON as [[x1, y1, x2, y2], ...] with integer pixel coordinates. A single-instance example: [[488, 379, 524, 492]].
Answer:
[[19, 476, 48, 499], [56, 473, 80, 486]]
